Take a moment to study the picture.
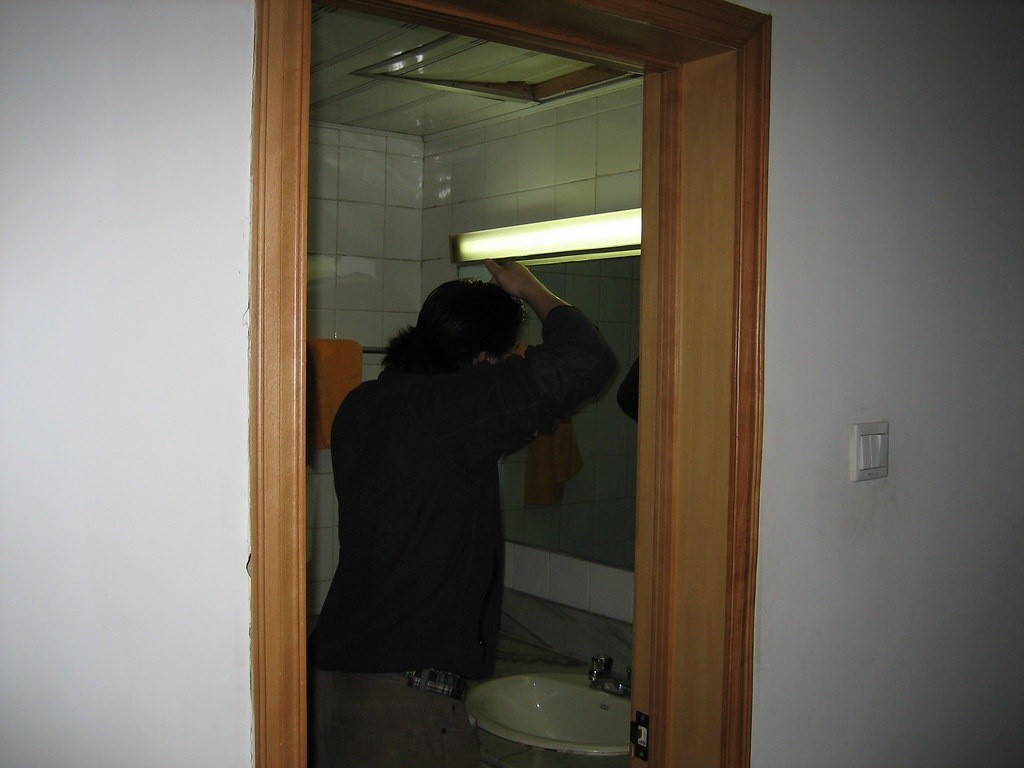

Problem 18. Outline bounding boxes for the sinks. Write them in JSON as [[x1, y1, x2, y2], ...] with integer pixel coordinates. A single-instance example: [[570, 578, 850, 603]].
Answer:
[[458, 673, 629, 768]]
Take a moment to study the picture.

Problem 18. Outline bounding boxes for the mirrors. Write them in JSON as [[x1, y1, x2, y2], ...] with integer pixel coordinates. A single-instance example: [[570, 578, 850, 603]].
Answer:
[[456, 248, 641, 571]]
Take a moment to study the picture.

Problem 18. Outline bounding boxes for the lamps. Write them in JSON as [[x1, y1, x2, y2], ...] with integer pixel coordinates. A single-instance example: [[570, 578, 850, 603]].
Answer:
[[448, 207, 642, 264]]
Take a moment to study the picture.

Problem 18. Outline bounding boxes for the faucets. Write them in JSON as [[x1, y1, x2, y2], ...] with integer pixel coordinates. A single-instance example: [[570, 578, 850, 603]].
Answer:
[[587, 653, 631, 698]]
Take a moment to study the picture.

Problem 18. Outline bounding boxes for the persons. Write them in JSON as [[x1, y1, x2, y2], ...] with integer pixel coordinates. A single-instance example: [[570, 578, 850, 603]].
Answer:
[[306, 259, 617, 768], [617, 355, 639, 423]]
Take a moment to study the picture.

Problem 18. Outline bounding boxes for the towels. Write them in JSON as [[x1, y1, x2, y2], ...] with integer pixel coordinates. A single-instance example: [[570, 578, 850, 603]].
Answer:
[[516, 345, 585, 511], [307, 339, 362, 470]]
